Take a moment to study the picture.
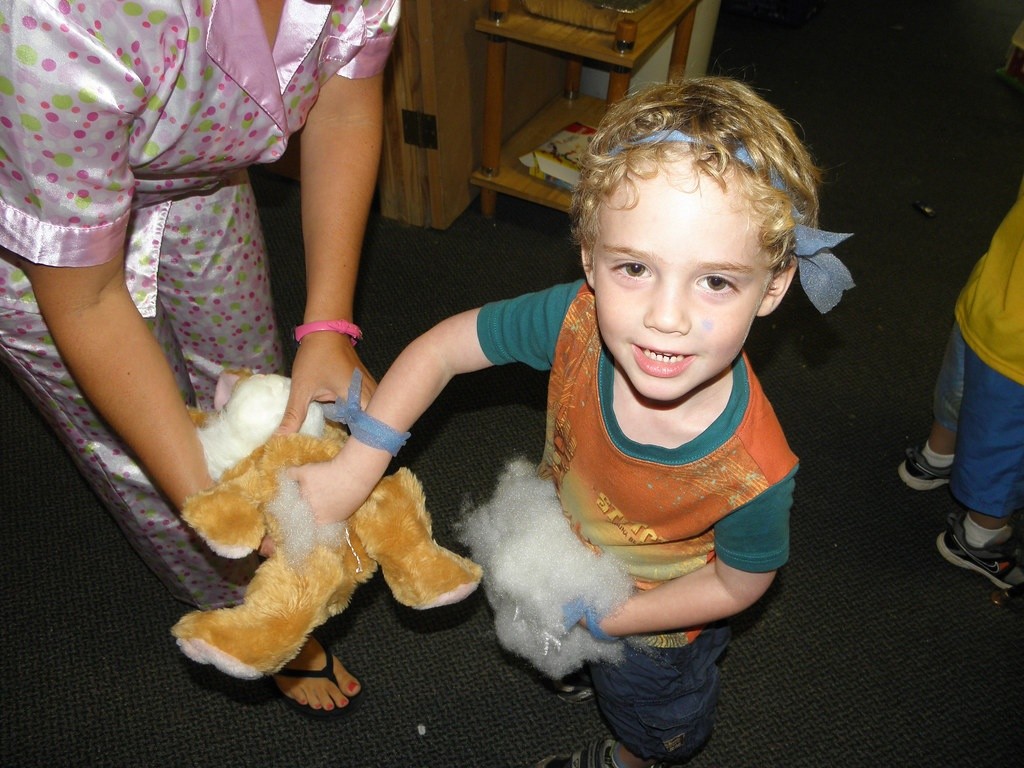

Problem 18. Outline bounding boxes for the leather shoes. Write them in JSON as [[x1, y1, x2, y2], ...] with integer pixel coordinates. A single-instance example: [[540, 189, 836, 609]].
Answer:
[[991, 580, 1024, 611]]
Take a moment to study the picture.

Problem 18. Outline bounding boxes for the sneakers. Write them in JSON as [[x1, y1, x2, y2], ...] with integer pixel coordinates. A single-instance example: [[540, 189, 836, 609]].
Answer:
[[535, 739, 663, 768], [557, 682, 595, 705], [936, 512, 1020, 589], [898, 444, 954, 491]]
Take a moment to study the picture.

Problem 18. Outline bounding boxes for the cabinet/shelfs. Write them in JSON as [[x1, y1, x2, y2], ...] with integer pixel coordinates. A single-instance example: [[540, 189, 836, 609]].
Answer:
[[468, 0, 702, 217]]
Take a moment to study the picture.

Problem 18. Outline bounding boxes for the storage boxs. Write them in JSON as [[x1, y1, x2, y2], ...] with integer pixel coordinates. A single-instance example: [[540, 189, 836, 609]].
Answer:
[[519, 121, 598, 189]]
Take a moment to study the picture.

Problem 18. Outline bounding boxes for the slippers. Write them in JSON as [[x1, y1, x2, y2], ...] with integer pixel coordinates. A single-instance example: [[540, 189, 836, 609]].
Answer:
[[273, 630, 367, 722]]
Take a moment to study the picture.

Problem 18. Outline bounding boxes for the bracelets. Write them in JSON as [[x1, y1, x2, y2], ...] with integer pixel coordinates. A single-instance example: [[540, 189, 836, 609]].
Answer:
[[288, 318, 363, 349], [318, 369, 413, 458], [585, 610, 621, 644]]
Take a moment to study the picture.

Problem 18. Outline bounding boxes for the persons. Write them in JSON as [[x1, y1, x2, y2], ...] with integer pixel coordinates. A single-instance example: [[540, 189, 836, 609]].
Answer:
[[0, 0, 402, 718], [896, 174, 1024, 596], [285, 76, 858, 768]]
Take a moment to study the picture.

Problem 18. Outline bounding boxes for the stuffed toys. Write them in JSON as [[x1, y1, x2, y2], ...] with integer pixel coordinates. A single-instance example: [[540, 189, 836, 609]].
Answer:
[[170, 368, 482, 681]]
